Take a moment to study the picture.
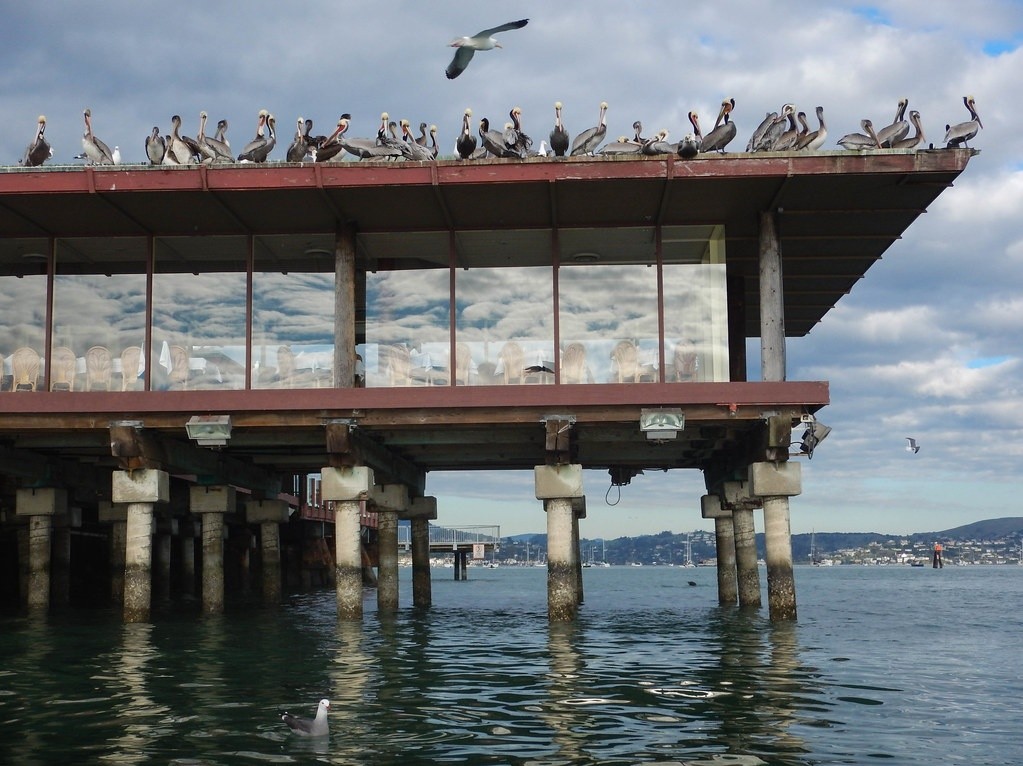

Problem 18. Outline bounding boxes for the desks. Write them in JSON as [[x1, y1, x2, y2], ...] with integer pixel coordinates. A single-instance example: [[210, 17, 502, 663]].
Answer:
[[190, 358, 221, 389], [77, 357, 122, 390], [277, 352, 364, 385], [493, 348, 590, 382], [610, 347, 698, 382], [4, 354, 44, 388], [384, 352, 478, 384]]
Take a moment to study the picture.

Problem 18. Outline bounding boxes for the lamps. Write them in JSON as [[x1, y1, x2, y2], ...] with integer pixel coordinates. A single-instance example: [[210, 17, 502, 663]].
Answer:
[[605, 467, 644, 485], [638, 405, 686, 441], [185, 414, 234, 447], [801, 423, 832, 456]]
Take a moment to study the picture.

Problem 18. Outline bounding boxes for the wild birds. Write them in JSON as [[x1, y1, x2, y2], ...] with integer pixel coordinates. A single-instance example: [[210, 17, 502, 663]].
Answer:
[[445, 18, 529, 80], [238, 111, 276, 163], [21, 114, 53, 167], [571, 102, 608, 157], [549, 102, 570, 156], [167, 115, 201, 165], [878, 95, 984, 148], [454, 110, 477, 161], [277, 698, 331, 736], [478, 108, 533, 158], [80, 108, 115, 165], [111, 146, 120, 165], [144, 127, 167, 166], [597, 111, 703, 157], [196, 111, 235, 164], [905, 437, 920, 454], [700, 98, 736, 153], [837, 121, 882, 149], [286, 113, 439, 163], [73, 152, 87, 160], [746, 103, 828, 153], [538, 140, 548, 157]]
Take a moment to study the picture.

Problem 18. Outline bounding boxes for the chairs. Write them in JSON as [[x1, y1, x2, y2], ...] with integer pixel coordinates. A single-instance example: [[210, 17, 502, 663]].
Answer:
[[389, 343, 411, 387], [667, 337, 698, 382], [85, 346, 113, 392], [164, 346, 187, 391], [502, 342, 526, 385], [11, 347, 39, 393], [277, 345, 296, 389], [561, 343, 586, 385], [615, 340, 657, 382], [449, 343, 470, 386], [121, 346, 146, 392], [50, 346, 75, 393]]
